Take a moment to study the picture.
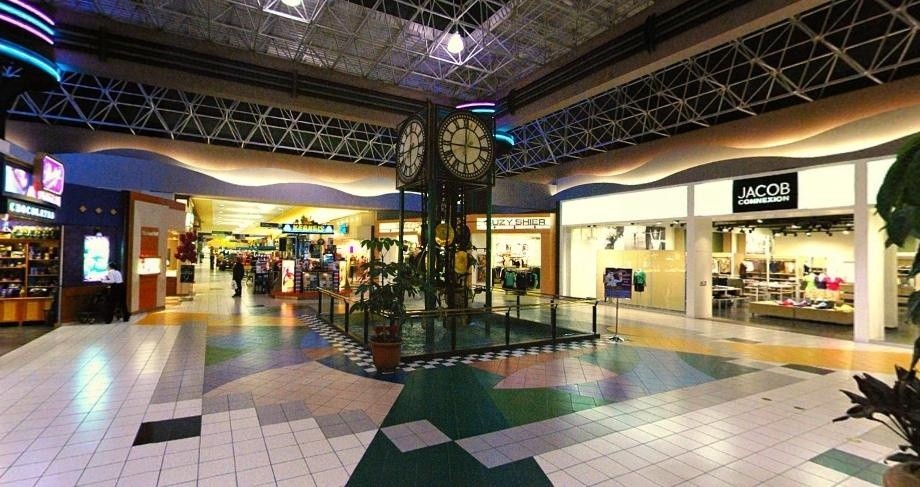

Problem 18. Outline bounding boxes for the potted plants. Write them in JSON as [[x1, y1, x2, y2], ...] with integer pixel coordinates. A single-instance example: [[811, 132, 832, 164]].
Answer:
[[348, 237, 423, 374], [832, 130, 919, 486]]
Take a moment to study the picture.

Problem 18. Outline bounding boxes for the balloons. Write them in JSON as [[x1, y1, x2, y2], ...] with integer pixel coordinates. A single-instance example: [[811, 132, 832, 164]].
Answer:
[[173, 230, 198, 264]]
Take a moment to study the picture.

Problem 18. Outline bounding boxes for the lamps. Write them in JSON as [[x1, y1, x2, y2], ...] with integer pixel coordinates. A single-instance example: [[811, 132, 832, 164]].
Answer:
[[281, 0, 300, 7], [447, 24, 464, 54]]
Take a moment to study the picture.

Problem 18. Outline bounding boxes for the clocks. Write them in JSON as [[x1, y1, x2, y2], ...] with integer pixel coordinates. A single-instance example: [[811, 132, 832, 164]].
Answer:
[[395, 114, 427, 185], [437, 112, 495, 182]]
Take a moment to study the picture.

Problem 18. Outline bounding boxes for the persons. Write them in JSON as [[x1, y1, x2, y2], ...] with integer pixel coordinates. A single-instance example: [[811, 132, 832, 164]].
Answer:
[[101, 259, 131, 324], [272, 259, 281, 279], [802, 272, 819, 298], [823, 268, 846, 299], [209, 245, 216, 270], [284, 267, 295, 292], [814, 272, 829, 298], [231, 256, 245, 298]]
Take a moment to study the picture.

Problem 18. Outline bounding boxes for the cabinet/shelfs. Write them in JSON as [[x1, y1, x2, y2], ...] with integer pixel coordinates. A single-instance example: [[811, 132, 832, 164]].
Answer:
[[715, 258, 805, 315], [0, 233, 61, 327]]
